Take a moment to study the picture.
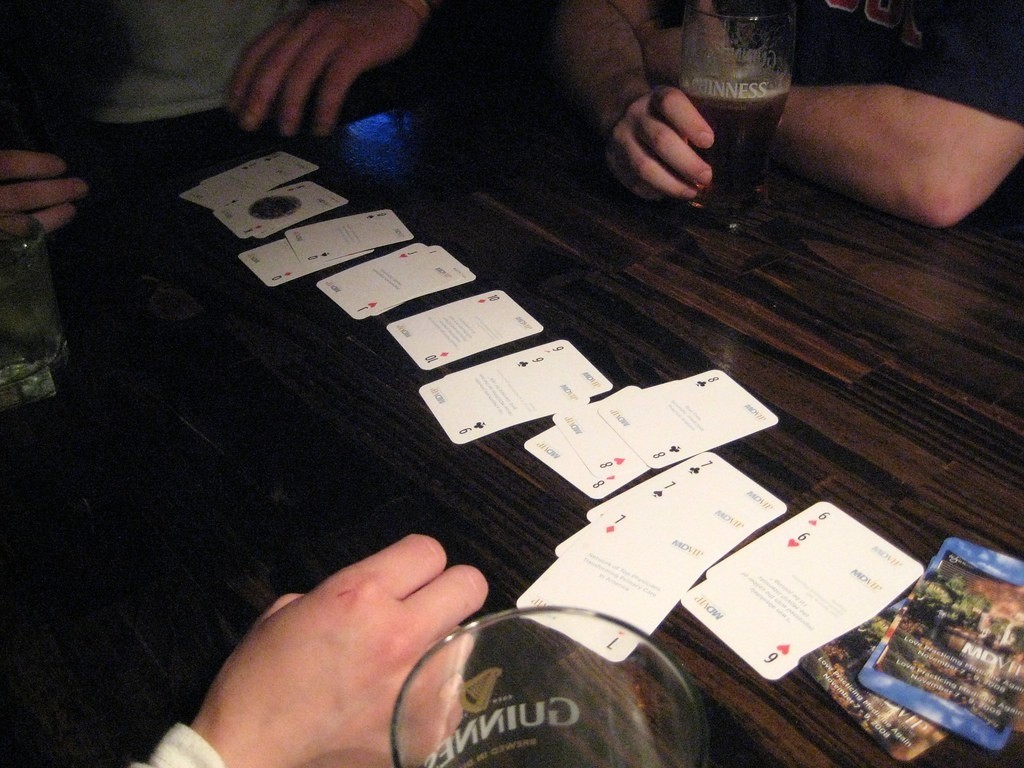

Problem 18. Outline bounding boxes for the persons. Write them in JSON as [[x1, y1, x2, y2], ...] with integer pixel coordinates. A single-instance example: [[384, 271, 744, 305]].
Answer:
[[554, 1, 1024, 230], [125, 531, 491, 767], [0, 0, 444, 238]]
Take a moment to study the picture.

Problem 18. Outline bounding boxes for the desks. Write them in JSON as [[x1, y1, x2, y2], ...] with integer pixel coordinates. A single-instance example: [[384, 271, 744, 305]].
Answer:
[[0, 125, 1024, 768]]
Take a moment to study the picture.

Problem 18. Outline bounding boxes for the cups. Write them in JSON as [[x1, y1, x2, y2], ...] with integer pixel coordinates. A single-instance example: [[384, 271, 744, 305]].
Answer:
[[390, 605, 710, 768], [0, 211, 73, 412], [680, 0, 798, 211]]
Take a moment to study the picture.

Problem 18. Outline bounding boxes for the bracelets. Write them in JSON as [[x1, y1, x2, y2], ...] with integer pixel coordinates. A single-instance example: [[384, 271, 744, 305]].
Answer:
[[422, 0, 436, 15]]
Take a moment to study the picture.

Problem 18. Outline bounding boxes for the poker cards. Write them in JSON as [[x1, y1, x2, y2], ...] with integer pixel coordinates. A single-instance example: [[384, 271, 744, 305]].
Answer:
[[377, 285, 544, 374], [174, 146, 1024, 765]]
